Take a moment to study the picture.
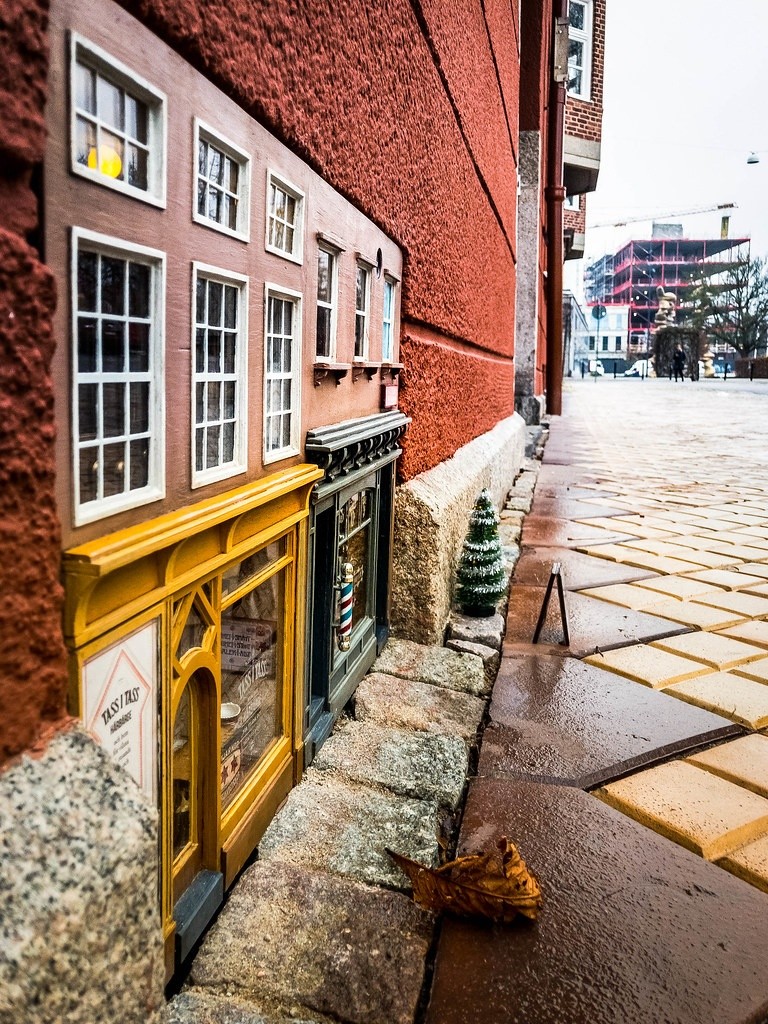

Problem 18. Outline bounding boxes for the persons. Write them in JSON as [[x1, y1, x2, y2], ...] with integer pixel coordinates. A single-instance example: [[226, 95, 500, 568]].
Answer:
[[673, 345, 686, 381]]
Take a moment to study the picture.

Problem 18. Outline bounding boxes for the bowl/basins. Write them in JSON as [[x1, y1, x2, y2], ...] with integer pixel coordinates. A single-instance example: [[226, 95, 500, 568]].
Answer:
[[221, 702, 241, 727]]
